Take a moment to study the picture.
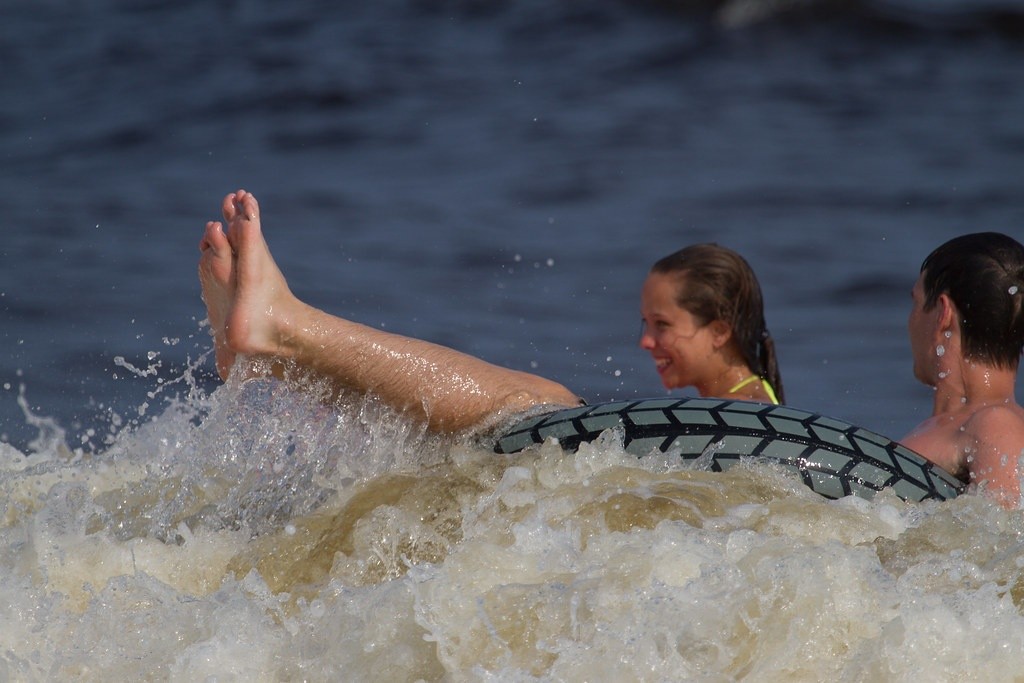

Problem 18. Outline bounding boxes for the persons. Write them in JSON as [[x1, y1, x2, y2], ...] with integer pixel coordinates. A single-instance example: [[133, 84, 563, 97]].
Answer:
[[200, 190, 1024, 512], [638, 241, 785, 406]]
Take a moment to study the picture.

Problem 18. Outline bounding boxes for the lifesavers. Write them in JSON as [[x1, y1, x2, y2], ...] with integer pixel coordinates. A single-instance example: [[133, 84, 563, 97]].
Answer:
[[494, 402, 966, 503]]
